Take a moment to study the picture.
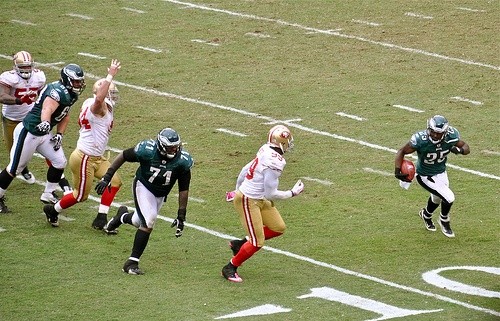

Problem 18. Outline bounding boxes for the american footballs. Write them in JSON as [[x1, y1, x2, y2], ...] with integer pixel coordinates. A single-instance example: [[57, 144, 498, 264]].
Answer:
[[400, 160, 415, 181]]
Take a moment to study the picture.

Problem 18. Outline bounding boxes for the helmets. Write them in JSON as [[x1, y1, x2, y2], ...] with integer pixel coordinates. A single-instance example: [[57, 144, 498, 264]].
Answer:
[[267, 125, 292, 152], [430, 115, 448, 133], [157, 127, 181, 146], [93, 78, 120, 93], [13, 51, 32, 67], [60, 63, 84, 90]]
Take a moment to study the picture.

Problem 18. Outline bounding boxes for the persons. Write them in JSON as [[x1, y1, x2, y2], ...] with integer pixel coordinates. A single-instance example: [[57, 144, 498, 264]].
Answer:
[[222, 125, 304, 283], [395, 115, 470, 239], [0, 51, 73, 195], [0, 64, 87, 213], [44, 59, 121, 233], [95, 128, 194, 274]]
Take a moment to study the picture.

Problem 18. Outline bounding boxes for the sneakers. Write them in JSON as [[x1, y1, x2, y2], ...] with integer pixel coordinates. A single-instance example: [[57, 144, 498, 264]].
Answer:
[[122, 257, 145, 275], [0, 195, 12, 213], [40, 191, 61, 205], [221, 262, 244, 283], [106, 205, 128, 231], [43, 205, 60, 226], [419, 207, 456, 238], [91, 212, 118, 235], [230, 235, 250, 256]]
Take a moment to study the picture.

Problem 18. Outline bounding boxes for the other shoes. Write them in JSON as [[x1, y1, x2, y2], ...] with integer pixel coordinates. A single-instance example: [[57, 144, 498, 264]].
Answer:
[[59, 177, 74, 196], [21, 166, 36, 184]]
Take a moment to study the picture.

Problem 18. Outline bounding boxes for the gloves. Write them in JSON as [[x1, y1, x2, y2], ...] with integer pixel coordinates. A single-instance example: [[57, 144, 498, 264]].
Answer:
[[170, 208, 187, 238], [449, 146, 464, 155], [34, 120, 51, 135], [395, 168, 412, 183], [292, 179, 304, 196], [20, 90, 37, 105], [225, 190, 236, 201], [50, 133, 64, 151], [95, 167, 115, 195]]
